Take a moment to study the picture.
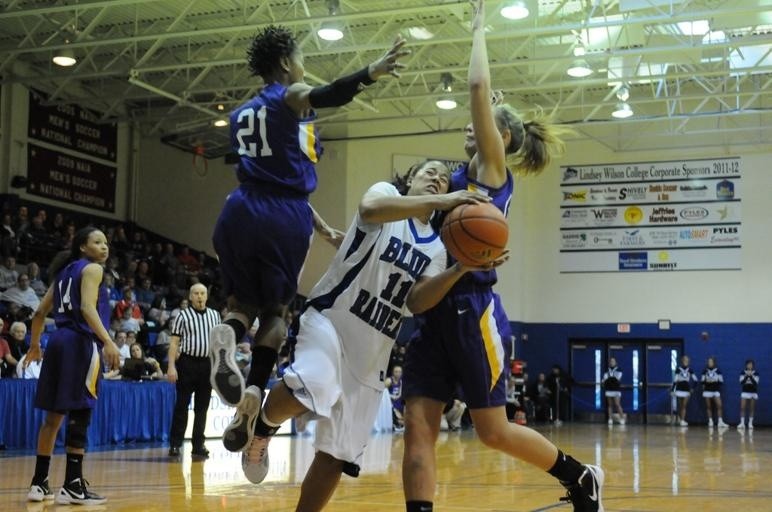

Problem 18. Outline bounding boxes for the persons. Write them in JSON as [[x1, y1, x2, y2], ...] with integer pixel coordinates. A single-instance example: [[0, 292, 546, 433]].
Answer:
[[402, 1, 603, 512], [241, 324, 258, 350], [136, 262, 148, 287], [0, 319, 19, 380], [104, 270, 119, 300], [700, 357, 729, 429], [51, 212, 61, 230], [166, 282, 222, 458], [504, 377, 520, 419], [108, 316, 121, 338], [212, 26, 410, 452], [121, 273, 137, 300], [0, 213, 16, 239], [112, 285, 144, 325], [736, 360, 759, 431], [530, 372, 546, 422], [127, 331, 136, 347], [126, 261, 137, 273], [38, 209, 46, 221], [148, 295, 169, 325], [139, 278, 155, 310], [8, 322, 29, 361], [24, 216, 46, 234], [120, 307, 140, 334], [151, 242, 164, 262], [103, 332, 131, 367], [15, 341, 43, 379], [220, 306, 228, 316], [6, 302, 20, 323], [130, 232, 145, 251], [22, 227, 123, 506], [239, 158, 510, 512], [107, 255, 119, 280], [385, 366, 405, 432], [12, 206, 30, 232], [544, 365, 566, 424], [669, 355, 698, 428], [162, 243, 179, 268], [444, 399, 465, 431], [178, 245, 196, 264], [58, 225, 76, 250], [171, 299, 188, 317], [601, 356, 628, 426], [156, 316, 174, 344], [0, 255, 19, 291], [130, 343, 164, 378], [3, 273, 40, 320], [26, 262, 47, 296]]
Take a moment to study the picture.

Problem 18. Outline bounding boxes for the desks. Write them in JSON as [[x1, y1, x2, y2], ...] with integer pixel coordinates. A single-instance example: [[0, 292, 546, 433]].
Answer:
[[0, 377, 177, 452], [184, 387, 295, 439], [304, 389, 393, 433]]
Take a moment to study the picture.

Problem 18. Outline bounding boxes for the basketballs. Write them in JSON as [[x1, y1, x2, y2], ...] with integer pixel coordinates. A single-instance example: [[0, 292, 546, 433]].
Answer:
[[440, 200, 510, 266]]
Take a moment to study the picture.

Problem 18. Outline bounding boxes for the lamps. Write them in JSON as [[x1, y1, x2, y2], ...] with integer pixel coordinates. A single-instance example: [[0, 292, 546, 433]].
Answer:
[[54, 47, 77, 67], [612, 103, 633, 118], [502, 3, 529, 19], [214, 114, 227, 127], [436, 89, 458, 110], [318, 19, 345, 41], [566, 58, 591, 76]]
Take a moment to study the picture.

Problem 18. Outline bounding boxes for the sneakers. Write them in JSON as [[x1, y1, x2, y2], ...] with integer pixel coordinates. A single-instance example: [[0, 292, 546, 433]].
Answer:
[[680, 420, 688, 426], [737, 428, 745, 433], [608, 419, 613, 424], [737, 423, 745, 428], [28, 477, 55, 501], [208, 323, 246, 408], [169, 446, 180, 456], [241, 430, 272, 485], [222, 384, 267, 453], [748, 424, 754, 429], [191, 446, 209, 455], [620, 418, 625, 424], [708, 421, 713, 426], [560, 463, 606, 512], [717, 421, 729, 427], [718, 428, 730, 432], [57, 484, 108, 505]]
